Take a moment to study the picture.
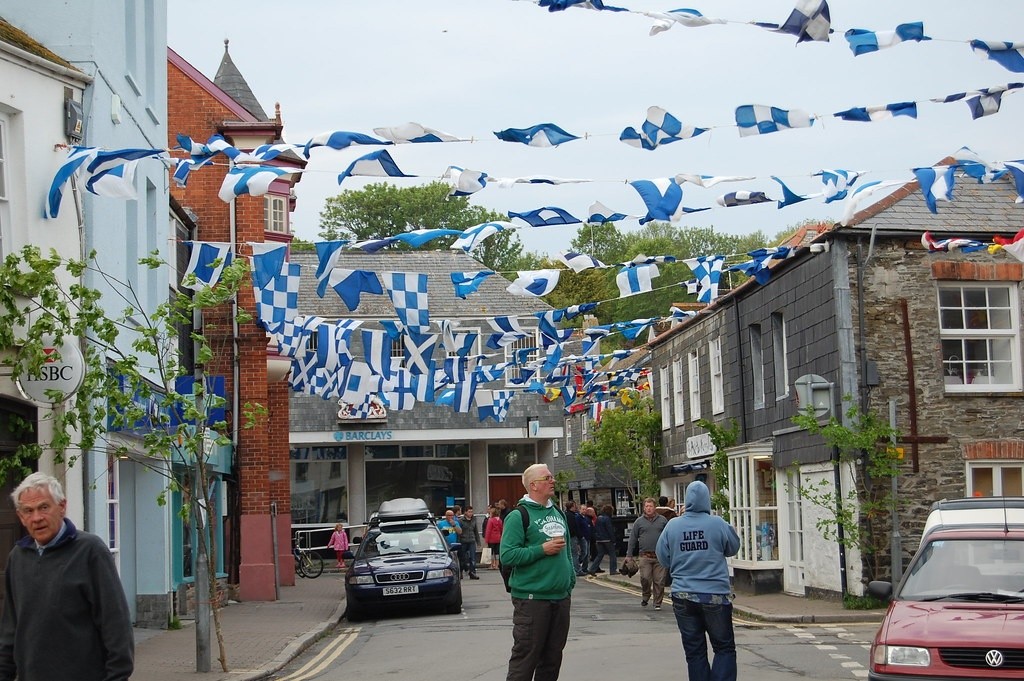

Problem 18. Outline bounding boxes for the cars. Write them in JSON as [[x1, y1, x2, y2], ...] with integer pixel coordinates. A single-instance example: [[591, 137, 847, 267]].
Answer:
[[865, 524, 1024, 681], [916, 494, 1023, 577]]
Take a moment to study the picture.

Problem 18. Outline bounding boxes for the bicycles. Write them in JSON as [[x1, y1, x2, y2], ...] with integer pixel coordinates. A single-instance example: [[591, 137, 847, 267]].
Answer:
[[291, 537, 325, 579]]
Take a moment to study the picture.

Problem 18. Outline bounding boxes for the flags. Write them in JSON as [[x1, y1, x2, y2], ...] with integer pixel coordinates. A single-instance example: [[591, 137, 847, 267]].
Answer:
[[44, 0, 1024, 423]]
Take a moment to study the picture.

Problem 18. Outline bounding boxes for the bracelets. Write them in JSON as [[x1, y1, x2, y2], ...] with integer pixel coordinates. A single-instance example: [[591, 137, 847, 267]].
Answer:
[[453, 525, 456, 527]]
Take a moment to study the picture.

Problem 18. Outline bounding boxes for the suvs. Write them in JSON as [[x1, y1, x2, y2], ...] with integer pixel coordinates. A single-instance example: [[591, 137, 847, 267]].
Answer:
[[343, 497, 463, 622]]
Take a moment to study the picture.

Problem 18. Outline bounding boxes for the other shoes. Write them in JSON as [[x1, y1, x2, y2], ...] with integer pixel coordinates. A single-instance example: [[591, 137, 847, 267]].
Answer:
[[641, 600, 647, 606], [470, 574, 479, 579], [335, 564, 341, 567], [610, 571, 620, 575], [340, 564, 345, 567], [595, 568, 605, 572], [654, 605, 660, 610], [576, 571, 587, 576], [588, 570, 597, 576]]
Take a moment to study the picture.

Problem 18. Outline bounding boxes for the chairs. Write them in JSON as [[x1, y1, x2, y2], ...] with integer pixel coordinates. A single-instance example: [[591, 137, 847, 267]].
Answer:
[[945, 565, 983, 590], [376, 538, 394, 553], [418, 533, 433, 550]]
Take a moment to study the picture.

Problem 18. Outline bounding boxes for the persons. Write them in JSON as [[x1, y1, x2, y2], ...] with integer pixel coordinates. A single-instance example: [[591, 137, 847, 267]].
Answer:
[[437, 505, 481, 580], [564, 500, 605, 576], [655, 496, 679, 521], [327, 523, 348, 567], [500, 463, 577, 681], [655, 481, 741, 681], [625, 498, 669, 610], [0, 472, 135, 681], [481, 499, 511, 570], [587, 505, 620, 577]]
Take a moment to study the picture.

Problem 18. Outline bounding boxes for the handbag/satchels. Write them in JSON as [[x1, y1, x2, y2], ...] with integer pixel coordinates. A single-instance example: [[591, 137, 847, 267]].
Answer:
[[480, 545, 492, 565]]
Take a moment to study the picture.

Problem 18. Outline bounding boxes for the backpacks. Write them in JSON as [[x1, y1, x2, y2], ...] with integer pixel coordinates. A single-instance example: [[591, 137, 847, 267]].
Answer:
[[498, 505, 565, 593]]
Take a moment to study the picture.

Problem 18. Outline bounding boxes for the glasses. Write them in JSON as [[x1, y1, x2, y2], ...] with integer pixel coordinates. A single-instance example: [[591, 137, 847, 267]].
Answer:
[[531, 476, 554, 483]]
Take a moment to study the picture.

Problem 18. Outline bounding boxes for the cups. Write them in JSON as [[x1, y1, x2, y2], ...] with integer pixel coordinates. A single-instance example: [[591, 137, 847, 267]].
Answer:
[[552, 532, 565, 542]]
